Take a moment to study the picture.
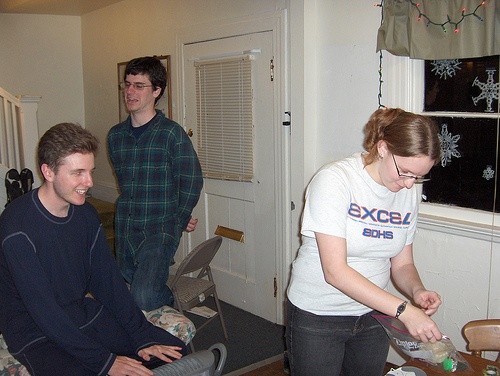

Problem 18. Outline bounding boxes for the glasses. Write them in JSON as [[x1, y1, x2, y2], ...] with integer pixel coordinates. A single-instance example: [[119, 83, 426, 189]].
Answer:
[[124, 81, 153, 90], [391, 152, 431, 184]]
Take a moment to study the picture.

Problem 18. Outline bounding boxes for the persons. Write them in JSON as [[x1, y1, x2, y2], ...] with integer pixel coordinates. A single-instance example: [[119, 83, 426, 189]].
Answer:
[[285, 109, 442, 376], [0, 123, 190, 376], [107, 56, 204, 312]]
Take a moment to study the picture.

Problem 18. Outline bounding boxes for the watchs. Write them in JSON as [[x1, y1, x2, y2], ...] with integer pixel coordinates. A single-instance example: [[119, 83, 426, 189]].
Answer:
[[395, 301, 407, 318]]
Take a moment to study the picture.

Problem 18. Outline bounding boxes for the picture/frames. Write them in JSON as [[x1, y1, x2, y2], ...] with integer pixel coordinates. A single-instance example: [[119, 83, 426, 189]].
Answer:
[[117, 54, 172, 123]]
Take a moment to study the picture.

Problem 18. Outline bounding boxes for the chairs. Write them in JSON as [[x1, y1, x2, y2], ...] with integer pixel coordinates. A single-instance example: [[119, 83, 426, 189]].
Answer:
[[166, 236, 228, 354], [462, 319, 500, 363]]
